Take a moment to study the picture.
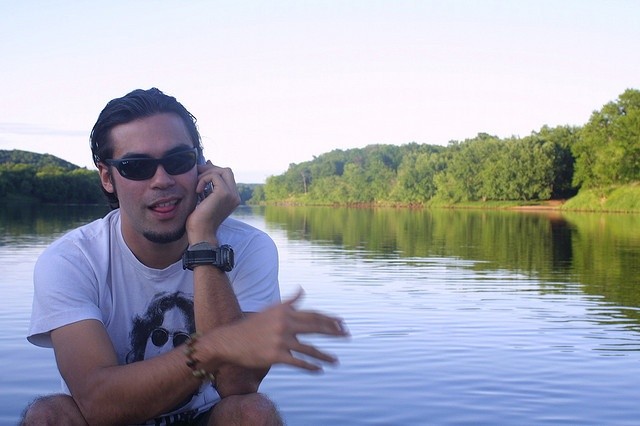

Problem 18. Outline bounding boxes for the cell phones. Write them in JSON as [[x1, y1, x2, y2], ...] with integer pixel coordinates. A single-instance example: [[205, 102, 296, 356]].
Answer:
[[199, 154, 212, 195]]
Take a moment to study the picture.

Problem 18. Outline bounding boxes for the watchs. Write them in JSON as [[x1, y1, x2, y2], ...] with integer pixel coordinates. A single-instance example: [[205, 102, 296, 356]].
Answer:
[[182, 241, 235, 273]]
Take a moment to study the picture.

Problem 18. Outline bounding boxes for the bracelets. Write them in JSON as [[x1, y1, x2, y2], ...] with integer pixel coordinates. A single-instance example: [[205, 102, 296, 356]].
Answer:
[[185, 332, 217, 381]]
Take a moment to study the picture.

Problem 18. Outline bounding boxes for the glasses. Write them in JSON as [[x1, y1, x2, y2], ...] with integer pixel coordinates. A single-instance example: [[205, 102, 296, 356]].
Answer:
[[105, 147, 199, 181]]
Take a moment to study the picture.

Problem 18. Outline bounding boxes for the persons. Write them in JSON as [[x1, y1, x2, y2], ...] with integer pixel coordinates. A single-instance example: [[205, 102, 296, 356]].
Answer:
[[20, 88, 352, 426], [126, 291, 202, 413]]
